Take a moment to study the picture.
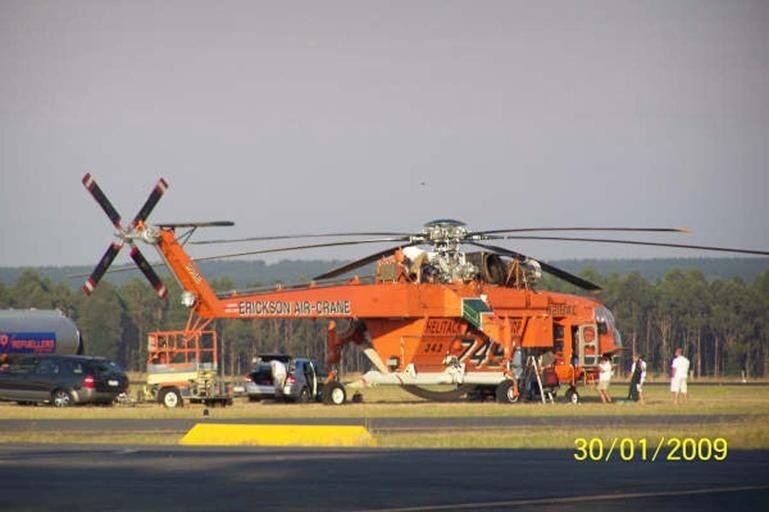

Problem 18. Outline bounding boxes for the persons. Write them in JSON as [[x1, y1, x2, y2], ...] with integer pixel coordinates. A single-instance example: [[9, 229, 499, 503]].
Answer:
[[670, 349, 690, 403], [628, 353, 647, 405], [403, 246, 434, 282], [739, 364, 747, 381], [596, 357, 612, 402], [271, 360, 287, 392]]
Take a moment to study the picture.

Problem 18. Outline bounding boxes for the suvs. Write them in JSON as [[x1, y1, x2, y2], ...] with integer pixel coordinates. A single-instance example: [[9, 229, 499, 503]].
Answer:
[[1, 352, 129, 408]]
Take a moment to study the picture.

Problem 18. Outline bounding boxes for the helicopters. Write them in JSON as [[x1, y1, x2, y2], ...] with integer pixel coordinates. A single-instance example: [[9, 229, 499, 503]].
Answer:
[[74, 170, 769, 405]]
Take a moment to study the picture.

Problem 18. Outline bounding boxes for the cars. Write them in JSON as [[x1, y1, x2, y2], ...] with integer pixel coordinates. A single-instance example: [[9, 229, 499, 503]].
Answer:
[[243, 350, 338, 403]]
